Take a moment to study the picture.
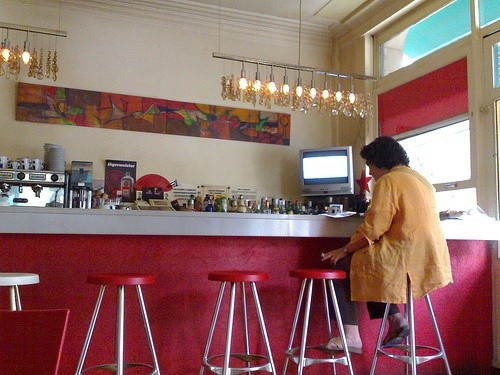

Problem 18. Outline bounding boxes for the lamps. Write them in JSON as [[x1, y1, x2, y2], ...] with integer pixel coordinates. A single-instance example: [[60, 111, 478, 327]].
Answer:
[[0, 21, 67, 81], [213, 0, 377, 119]]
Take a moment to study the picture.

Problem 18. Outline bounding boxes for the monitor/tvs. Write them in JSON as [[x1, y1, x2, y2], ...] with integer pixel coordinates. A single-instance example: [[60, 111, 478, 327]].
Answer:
[[300, 145, 354, 203]]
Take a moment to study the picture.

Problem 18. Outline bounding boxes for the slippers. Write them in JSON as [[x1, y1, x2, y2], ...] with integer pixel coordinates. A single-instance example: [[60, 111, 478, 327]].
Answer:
[[382, 322, 409, 347], [319, 337, 362, 355]]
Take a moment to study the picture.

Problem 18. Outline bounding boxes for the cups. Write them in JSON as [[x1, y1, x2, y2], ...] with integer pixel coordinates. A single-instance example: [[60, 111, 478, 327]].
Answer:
[[92, 196, 122, 208], [0, 156, 44, 171]]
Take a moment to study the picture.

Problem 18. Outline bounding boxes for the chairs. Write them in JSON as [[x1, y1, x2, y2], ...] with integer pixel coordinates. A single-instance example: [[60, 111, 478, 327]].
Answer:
[[0, 308, 70, 375]]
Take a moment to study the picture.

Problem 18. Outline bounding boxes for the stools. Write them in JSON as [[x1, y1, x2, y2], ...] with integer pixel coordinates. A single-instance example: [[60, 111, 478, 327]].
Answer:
[[198, 271, 276, 375], [281, 269, 354, 375], [0, 273, 40, 311], [75, 272, 161, 375], [370, 276, 451, 375]]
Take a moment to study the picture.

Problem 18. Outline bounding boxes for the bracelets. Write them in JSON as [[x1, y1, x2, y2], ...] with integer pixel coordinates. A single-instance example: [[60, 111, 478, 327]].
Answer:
[[344, 244, 350, 254]]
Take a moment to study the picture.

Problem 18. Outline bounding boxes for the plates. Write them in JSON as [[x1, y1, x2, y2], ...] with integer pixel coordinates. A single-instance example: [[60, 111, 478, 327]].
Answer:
[[43, 143, 66, 172]]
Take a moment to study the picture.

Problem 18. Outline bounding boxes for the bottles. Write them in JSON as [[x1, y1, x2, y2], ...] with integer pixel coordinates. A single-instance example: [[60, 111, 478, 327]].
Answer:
[[187, 194, 313, 215]]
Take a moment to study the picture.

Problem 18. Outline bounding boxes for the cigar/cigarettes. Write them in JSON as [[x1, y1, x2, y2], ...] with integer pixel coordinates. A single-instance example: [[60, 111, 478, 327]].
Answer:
[[322, 252, 325, 258]]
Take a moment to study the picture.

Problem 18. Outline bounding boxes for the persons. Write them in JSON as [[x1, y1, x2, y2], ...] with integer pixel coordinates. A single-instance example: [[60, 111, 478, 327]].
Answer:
[[320, 135, 452, 355]]
